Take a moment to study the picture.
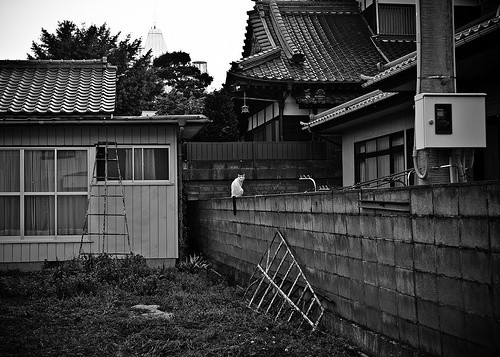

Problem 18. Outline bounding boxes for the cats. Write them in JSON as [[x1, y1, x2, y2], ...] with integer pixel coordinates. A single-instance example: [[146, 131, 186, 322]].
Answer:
[[229, 173, 245, 198]]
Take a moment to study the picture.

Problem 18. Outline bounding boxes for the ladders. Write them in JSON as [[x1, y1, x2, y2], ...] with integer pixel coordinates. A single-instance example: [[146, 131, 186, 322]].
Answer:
[[239, 228, 325, 338], [77, 141, 134, 258]]
